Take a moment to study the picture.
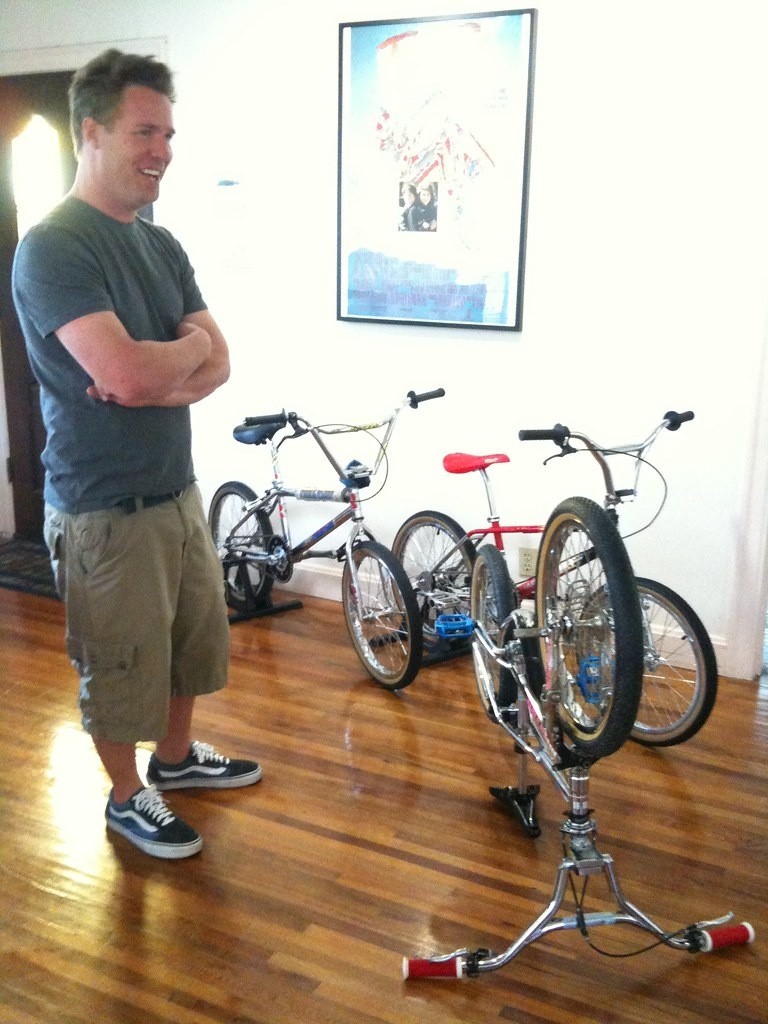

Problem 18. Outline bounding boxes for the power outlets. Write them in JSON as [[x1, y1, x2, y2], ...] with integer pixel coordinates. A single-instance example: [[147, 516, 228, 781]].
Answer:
[[518, 547, 538, 576]]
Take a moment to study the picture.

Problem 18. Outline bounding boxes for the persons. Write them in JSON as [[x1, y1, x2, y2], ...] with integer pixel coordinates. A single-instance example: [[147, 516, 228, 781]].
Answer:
[[11, 49, 261, 862], [399, 183, 437, 231]]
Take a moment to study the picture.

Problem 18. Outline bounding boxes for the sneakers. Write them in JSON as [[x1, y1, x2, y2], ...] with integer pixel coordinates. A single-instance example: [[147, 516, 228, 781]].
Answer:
[[146, 740, 263, 791], [105, 784, 204, 859]]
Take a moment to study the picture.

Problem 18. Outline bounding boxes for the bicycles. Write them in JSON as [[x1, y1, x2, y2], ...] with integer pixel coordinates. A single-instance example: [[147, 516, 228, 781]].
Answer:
[[387, 411, 718, 747], [206, 387, 446, 692], [401, 497, 756, 983]]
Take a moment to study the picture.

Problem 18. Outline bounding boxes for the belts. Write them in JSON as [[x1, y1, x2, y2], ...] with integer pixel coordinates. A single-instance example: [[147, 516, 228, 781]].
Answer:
[[116, 487, 186, 515]]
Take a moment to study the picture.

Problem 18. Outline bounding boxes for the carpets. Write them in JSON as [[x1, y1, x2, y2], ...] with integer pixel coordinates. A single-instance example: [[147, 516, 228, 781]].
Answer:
[[0, 536, 59, 600]]
